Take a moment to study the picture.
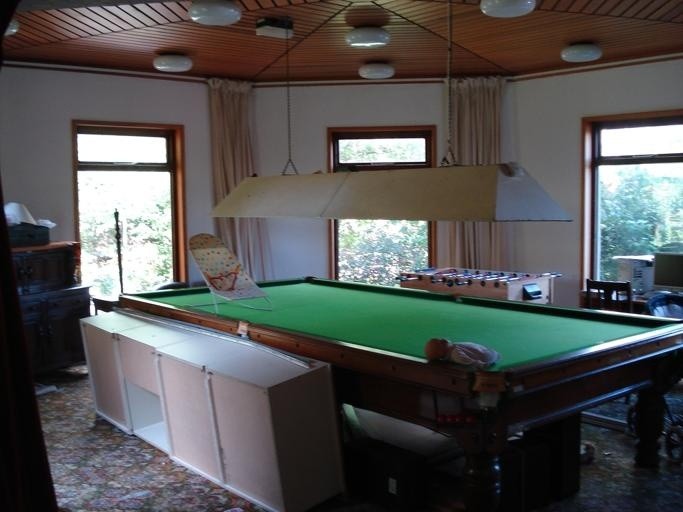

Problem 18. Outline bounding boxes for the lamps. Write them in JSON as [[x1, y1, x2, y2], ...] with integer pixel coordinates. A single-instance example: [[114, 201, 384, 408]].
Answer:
[[207, 13, 575, 226]]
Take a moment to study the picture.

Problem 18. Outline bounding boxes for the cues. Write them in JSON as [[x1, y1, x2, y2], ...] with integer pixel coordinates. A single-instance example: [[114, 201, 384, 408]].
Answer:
[[114, 306, 311, 369]]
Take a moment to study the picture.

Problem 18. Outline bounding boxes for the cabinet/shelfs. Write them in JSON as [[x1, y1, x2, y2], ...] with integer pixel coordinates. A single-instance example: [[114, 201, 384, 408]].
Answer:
[[116, 322, 224, 455], [157, 336, 348, 507], [79, 311, 175, 435], [7, 241, 92, 381]]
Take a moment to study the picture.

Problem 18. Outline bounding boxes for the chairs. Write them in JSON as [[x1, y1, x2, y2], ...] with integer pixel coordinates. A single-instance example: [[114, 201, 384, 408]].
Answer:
[[584, 275, 634, 313]]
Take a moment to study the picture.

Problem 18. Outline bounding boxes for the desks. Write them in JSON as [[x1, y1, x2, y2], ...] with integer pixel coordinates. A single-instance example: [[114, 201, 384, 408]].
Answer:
[[118, 275, 683, 507]]
